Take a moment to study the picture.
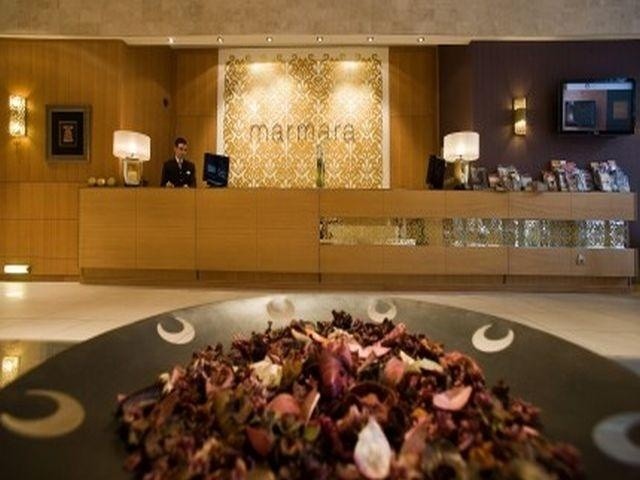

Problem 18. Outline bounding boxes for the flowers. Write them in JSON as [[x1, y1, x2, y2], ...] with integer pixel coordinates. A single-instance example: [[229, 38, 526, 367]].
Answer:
[[110, 306, 588, 480]]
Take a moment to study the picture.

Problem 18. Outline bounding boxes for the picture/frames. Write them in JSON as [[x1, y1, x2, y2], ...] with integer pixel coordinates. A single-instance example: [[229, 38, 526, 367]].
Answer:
[[44, 104, 92, 165]]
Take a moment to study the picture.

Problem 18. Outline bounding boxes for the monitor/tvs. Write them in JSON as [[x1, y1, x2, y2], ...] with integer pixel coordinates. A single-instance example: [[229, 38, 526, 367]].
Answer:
[[202, 152, 229, 186], [426, 154, 446, 190]]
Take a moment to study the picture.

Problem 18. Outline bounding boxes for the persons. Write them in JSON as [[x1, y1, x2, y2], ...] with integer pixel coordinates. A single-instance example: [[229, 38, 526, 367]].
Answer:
[[160, 137, 196, 188]]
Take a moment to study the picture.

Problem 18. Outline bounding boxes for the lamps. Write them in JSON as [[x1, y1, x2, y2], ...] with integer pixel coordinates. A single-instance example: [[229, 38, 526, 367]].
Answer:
[[113, 128, 152, 184], [442, 131, 479, 190], [510, 97, 530, 136], [8, 94, 27, 137]]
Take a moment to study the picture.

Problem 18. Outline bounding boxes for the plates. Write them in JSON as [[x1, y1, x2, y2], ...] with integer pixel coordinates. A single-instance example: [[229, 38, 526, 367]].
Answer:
[[1, 292, 640, 479]]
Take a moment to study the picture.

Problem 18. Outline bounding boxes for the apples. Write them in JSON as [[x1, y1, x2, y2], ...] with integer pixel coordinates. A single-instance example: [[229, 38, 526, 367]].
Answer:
[[97, 178, 106, 186], [88, 176, 96, 186], [107, 177, 115, 187]]
